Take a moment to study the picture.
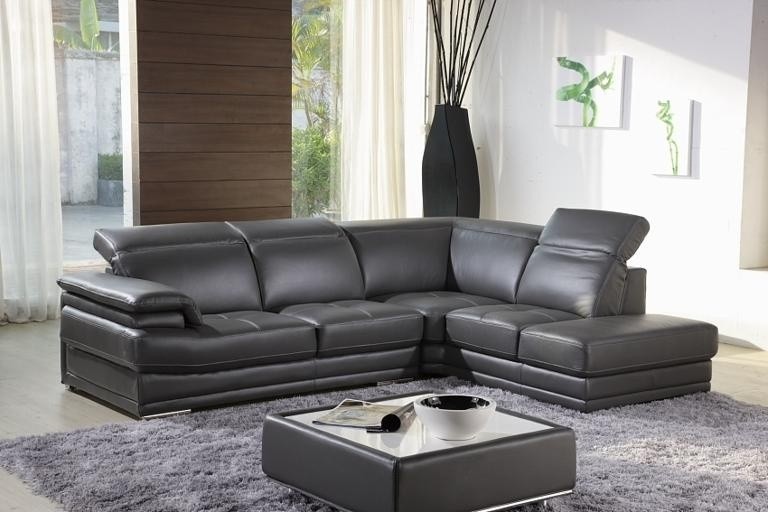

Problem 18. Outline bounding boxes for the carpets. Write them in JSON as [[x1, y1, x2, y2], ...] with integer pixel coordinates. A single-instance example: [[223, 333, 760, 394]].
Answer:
[[1, 376, 762, 512]]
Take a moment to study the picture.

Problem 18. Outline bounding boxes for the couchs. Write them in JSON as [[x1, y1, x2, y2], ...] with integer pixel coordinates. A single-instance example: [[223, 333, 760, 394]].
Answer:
[[54, 208, 718, 421]]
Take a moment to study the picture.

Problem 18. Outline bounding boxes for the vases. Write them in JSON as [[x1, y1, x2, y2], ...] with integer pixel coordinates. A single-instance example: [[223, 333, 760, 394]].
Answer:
[[422, 102, 480, 219]]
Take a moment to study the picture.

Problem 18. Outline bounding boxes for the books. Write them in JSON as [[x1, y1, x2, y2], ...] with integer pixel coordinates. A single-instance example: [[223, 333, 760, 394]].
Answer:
[[312, 397, 418, 434]]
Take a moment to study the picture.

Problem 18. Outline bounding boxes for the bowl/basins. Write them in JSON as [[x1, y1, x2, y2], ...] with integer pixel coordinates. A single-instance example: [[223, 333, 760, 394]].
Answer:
[[413, 394, 495, 442]]
